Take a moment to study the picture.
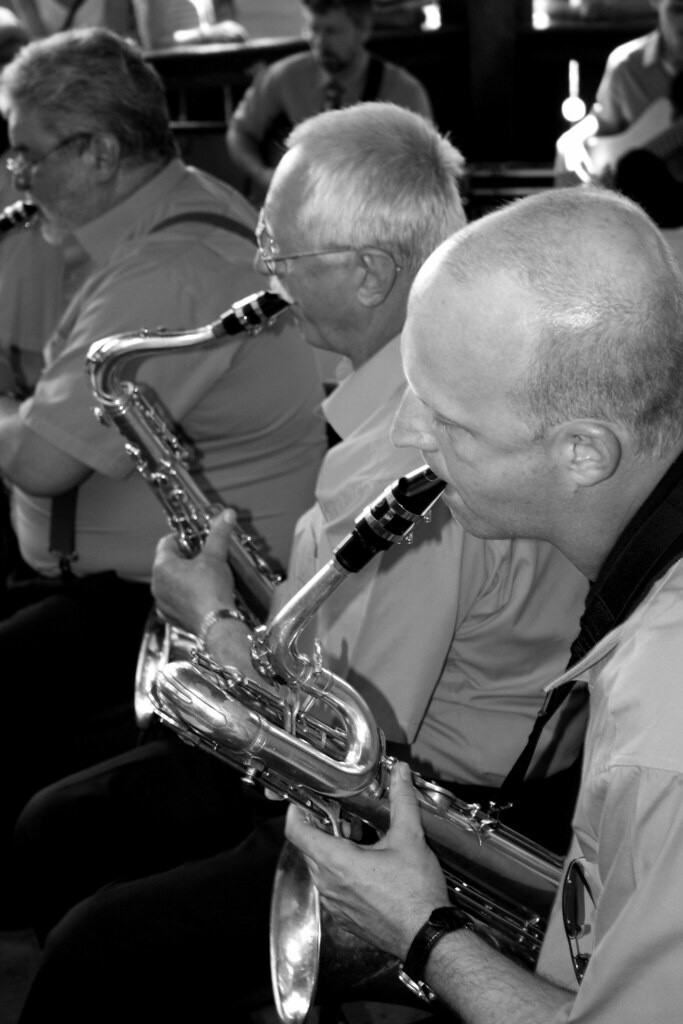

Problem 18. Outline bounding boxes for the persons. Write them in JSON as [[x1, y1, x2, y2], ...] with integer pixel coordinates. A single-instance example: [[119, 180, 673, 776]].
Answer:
[[3, 29, 328, 925], [285, 187, 683, 1024], [13, 103, 590, 1022], [2, 1, 130, 44], [593, 0, 681, 227], [226, 0, 435, 191]]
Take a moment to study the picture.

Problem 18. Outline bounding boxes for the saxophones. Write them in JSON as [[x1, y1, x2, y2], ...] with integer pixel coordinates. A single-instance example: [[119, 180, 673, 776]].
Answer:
[[83, 290, 291, 630], [137, 465, 570, 1023]]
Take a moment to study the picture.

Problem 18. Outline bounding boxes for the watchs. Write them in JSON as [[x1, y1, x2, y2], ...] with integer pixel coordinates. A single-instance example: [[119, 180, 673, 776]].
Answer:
[[398, 907, 478, 1003], [198, 607, 247, 649]]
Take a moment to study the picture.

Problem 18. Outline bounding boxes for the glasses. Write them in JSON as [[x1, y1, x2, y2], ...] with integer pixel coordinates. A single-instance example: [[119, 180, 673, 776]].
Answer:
[[256, 207, 405, 276], [6, 132, 95, 182]]
